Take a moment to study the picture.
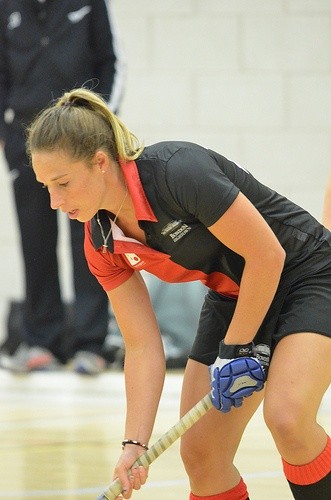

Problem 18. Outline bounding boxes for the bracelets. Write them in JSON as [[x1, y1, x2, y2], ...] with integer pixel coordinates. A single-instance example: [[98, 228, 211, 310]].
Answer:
[[121, 439, 149, 452]]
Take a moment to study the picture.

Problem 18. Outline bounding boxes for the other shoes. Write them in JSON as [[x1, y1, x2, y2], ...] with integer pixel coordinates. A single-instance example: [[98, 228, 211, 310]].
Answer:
[[23, 345, 66, 371], [72, 350, 108, 375]]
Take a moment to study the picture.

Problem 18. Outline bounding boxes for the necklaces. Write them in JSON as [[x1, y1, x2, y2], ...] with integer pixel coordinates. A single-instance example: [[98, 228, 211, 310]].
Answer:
[[96, 191, 128, 254]]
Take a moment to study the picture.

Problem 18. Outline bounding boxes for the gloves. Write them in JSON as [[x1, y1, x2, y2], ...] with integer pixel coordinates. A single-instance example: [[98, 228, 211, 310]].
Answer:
[[207, 339, 266, 413]]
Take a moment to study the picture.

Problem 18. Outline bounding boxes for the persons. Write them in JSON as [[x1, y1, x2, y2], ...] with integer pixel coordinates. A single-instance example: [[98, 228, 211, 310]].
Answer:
[[0, 0, 127, 375], [27, 88, 331, 500]]
